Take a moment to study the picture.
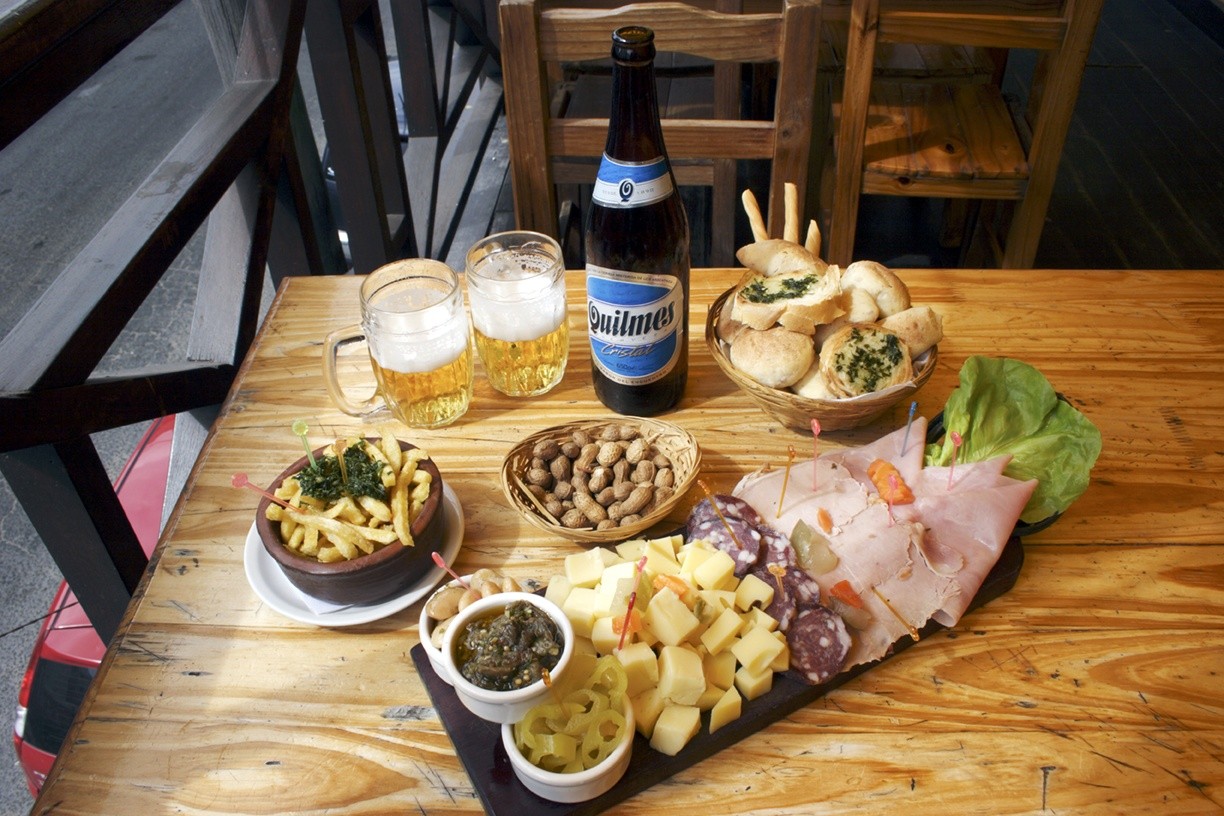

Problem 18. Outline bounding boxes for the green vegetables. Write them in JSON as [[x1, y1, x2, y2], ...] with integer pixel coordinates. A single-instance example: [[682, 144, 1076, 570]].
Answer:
[[921, 357, 1104, 528], [290, 439, 388, 501]]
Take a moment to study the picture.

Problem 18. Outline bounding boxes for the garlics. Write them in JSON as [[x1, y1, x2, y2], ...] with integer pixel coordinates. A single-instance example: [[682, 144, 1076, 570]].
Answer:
[[427, 567, 524, 653]]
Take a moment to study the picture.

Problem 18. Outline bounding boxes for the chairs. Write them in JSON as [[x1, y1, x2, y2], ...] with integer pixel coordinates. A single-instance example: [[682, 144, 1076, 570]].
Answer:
[[500, 0, 1102, 269]]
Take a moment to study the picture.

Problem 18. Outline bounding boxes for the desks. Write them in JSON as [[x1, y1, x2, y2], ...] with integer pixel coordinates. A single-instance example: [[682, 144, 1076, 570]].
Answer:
[[27, 266, 1224, 816]]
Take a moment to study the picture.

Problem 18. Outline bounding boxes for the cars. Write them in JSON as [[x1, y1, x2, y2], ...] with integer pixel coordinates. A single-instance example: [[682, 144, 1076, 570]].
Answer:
[[322, 53, 411, 268], [10, 414, 178, 798]]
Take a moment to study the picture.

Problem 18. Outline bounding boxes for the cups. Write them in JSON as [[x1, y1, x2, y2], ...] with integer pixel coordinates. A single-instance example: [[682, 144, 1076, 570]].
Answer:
[[321, 258, 474, 430], [464, 230, 570, 397]]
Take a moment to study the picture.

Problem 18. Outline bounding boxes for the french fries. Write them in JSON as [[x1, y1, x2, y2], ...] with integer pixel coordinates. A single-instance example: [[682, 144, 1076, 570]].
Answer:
[[265, 427, 431, 563]]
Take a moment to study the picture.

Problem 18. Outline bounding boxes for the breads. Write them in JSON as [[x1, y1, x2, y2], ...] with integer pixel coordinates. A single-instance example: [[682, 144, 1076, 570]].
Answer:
[[714, 183, 942, 402]]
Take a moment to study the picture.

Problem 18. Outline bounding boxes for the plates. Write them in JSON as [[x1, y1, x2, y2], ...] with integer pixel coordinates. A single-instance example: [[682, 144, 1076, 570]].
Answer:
[[243, 480, 466, 627], [411, 450, 1025, 816]]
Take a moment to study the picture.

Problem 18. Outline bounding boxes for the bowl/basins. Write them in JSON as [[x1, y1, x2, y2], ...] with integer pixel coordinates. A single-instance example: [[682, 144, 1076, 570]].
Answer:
[[501, 693, 637, 805], [445, 591, 574, 725], [255, 436, 444, 609], [418, 574, 473, 685]]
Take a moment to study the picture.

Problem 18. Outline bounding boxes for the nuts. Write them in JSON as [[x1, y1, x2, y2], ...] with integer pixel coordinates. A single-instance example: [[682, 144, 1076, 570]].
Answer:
[[518, 426, 676, 532]]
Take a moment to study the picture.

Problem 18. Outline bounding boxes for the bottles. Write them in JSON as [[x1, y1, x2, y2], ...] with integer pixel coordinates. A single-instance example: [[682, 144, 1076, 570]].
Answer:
[[584, 24, 693, 416]]
